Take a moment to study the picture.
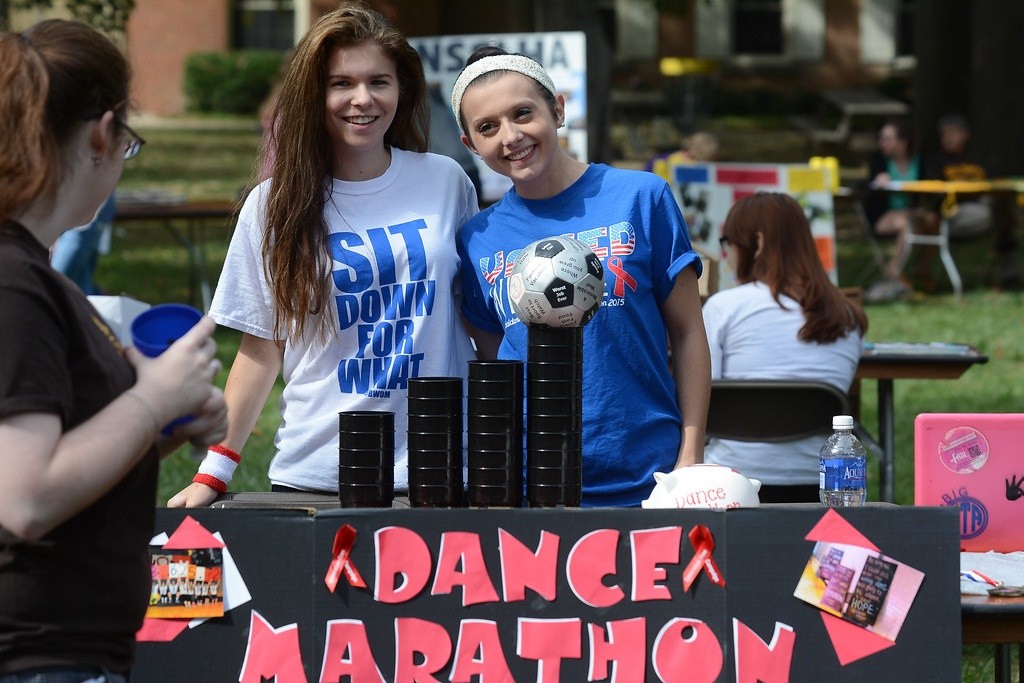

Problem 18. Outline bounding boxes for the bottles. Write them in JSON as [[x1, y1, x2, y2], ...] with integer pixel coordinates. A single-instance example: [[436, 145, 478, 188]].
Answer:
[[819, 415, 869, 508]]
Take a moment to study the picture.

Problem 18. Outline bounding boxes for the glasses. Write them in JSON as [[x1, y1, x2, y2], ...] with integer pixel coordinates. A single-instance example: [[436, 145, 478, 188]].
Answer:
[[719, 236, 729, 256], [84, 112, 146, 160]]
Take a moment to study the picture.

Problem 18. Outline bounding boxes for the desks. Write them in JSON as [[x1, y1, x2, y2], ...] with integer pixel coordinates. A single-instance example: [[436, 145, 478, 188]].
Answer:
[[879, 180, 1024, 300], [821, 85, 909, 151], [850, 342, 988, 502], [104, 199, 243, 319]]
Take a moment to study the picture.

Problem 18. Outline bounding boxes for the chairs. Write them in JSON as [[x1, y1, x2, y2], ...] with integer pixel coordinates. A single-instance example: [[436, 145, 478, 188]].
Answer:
[[704, 377, 852, 504], [847, 178, 898, 289]]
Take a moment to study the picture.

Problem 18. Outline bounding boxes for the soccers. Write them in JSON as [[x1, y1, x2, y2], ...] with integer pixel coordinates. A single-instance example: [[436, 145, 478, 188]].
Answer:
[[507, 234, 606, 328]]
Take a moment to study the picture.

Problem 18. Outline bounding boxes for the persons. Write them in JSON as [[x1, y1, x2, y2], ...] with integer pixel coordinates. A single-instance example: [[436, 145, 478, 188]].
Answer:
[[929, 118, 997, 293], [866, 122, 932, 304], [453, 47, 714, 509], [646, 127, 719, 186], [48, 196, 115, 297], [701, 191, 869, 507], [151, 547, 223, 608], [0, 19, 230, 683], [167, 8, 481, 507]]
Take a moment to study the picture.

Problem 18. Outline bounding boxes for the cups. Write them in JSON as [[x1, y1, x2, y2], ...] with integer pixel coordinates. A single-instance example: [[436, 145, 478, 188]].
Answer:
[[467, 358, 524, 510], [526, 323, 584, 507], [407, 375, 464, 510], [340, 409, 396, 509], [129, 304, 205, 438]]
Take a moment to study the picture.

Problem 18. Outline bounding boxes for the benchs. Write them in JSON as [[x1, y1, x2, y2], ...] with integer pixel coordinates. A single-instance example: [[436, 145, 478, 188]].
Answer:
[[788, 113, 841, 152]]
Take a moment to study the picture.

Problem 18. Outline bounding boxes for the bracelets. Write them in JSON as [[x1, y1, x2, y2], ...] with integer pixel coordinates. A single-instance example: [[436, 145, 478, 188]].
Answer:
[[125, 389, 160, 438], [192, 446, 241, 495]]
